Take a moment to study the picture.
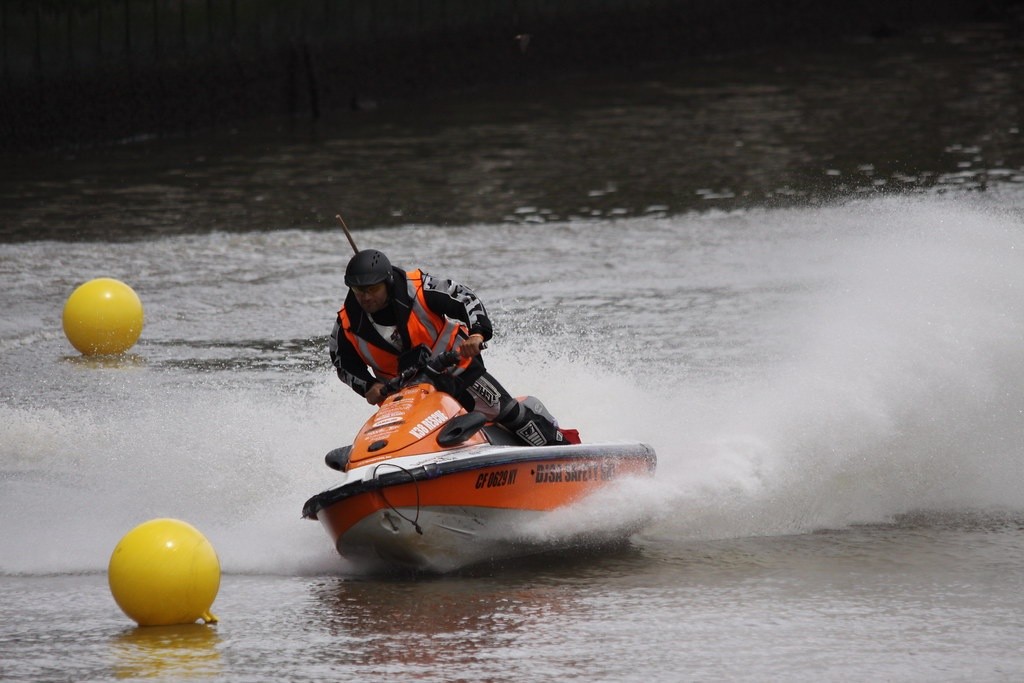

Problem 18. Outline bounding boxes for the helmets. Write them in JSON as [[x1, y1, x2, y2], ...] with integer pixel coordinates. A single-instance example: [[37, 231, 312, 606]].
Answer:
[[344, 249, 393, 287]]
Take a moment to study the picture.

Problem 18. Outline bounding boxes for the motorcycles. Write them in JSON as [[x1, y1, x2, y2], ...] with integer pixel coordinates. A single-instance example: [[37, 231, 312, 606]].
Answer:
[[302, 338, 659, 577]]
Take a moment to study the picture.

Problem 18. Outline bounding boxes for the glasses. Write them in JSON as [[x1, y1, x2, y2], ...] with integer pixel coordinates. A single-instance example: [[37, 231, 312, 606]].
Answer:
[[350, 280, 386, 296]]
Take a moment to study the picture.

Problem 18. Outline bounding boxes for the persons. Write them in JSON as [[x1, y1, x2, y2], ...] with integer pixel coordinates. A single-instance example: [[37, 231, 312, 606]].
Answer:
[[330, 249, 571, 447]]
